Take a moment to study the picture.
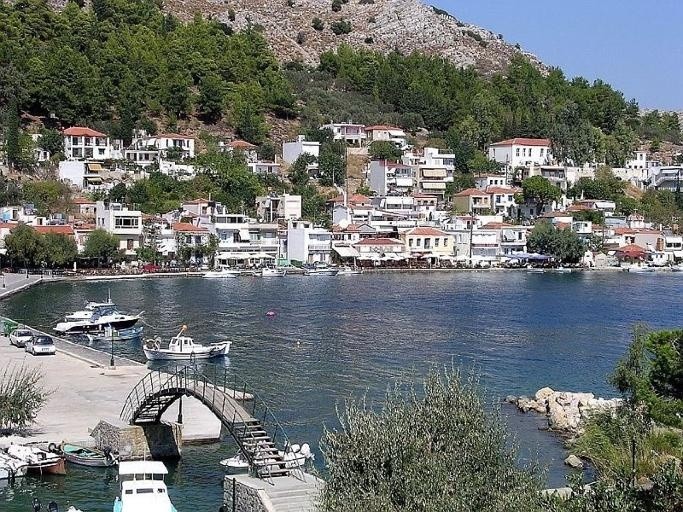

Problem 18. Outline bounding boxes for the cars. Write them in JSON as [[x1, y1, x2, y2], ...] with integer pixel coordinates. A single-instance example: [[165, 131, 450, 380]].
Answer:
[[24, 335, 55, 355], [9, 329, 33, 348]]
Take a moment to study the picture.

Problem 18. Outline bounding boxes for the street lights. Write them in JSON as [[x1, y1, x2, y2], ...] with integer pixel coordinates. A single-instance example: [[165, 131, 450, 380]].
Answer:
[[110, 327, 115, 366]]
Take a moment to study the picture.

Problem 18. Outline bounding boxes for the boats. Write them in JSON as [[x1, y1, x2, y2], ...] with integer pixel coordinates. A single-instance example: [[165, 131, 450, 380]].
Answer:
[[220, 443, 306, 473], [202, 264, 241, 278], [253, 264, 362, 277], [143, 325, 231, 360], [61, 441, 119, 466], [87, 326, 143, 341], [54, 299, 139, 334], [0, 445, 66, 479], [114, 461, 176, 512]]
